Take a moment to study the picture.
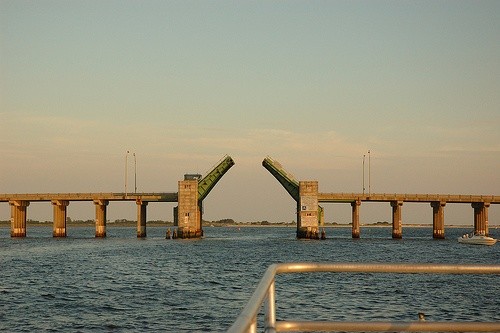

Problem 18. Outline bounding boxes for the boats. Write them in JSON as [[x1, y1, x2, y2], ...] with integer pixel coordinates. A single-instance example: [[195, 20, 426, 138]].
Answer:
[[457, 229, 498, 246]]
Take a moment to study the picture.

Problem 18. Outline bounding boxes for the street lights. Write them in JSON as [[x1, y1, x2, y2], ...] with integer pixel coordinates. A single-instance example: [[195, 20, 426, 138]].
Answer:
[[362, 150, 371, 197], [125, 150, 138, 197]]
